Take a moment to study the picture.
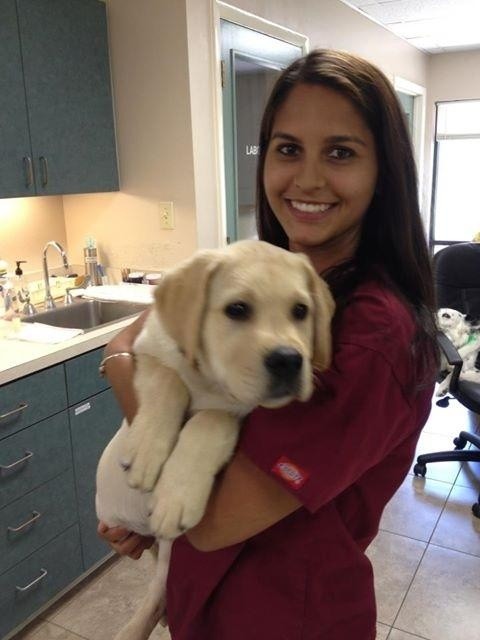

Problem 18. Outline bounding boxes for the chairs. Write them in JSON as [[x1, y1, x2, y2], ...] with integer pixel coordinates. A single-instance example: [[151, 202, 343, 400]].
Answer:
[[413, 242, 480, 518]]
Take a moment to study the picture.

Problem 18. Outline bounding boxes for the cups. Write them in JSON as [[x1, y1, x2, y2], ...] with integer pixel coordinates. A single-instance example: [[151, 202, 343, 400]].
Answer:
[[145, 274, 163, 285], [128, 271, 146, 284]]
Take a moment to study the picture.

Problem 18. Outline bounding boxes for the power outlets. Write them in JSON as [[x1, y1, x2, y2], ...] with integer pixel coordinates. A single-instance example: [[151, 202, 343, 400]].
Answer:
[[159, 202, 173, 228]]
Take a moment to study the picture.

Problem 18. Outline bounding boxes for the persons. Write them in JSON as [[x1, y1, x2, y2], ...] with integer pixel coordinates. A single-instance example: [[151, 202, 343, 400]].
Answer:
[[94, 48, 448, 640]]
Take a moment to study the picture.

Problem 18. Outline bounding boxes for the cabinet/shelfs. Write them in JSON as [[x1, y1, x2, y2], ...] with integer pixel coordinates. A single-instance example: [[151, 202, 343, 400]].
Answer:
[[0, 0, 119, 197], [63, 345, 126, 581], [0, 365, 82, 640]]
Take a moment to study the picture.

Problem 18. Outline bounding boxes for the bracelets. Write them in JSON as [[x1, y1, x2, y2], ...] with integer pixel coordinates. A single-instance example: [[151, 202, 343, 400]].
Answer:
[[97, 350, 134, 377]]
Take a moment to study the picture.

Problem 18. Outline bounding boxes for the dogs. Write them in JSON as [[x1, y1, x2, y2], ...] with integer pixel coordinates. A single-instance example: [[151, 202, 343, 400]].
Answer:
[[113, 238, 338, 640], [435, 307, 472, 398]]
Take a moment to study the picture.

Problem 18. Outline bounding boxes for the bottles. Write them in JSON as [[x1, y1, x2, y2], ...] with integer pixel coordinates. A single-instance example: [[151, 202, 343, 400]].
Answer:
[[0, 267, 20, 321], [83, 237, 99, 285]]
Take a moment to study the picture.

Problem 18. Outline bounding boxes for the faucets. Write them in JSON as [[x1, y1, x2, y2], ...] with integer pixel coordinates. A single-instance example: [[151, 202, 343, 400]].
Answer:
[[41, 240, 68, 309]]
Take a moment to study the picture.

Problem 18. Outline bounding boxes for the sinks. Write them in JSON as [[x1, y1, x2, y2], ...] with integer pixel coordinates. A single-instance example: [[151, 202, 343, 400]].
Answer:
[[0, 296, 156, 336]]
